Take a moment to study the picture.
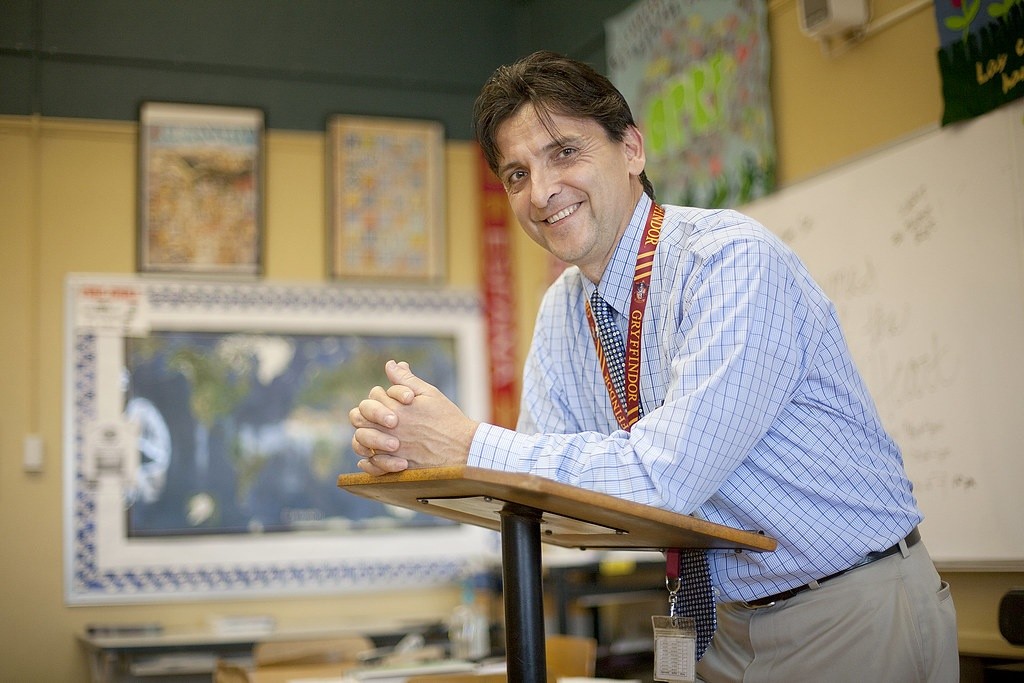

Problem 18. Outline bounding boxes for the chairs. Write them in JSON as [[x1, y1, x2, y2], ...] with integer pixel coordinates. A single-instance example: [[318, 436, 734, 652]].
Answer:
[[212, 655, 248, 683], [544, 634, 598, 683], [253, 632, 376, 665]]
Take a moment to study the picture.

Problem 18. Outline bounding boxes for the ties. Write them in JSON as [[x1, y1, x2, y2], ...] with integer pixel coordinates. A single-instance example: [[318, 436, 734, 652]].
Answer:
[[590, 290, 716, 661]]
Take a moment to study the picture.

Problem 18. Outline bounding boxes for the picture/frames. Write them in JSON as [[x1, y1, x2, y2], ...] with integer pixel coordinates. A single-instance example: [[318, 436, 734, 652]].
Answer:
[[135, 97, 267, 276], [63, 271, 502, 608], [323, 111, 450, 285]]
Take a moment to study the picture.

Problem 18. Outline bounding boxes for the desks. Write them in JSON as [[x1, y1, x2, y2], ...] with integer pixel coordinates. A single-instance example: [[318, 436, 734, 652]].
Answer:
[[241, 657, 509, 682], [69, 610, 498, 683]]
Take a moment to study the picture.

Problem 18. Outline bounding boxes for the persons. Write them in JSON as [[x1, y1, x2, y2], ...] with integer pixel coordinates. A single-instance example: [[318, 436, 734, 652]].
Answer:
[[346, 50, 960, 683]]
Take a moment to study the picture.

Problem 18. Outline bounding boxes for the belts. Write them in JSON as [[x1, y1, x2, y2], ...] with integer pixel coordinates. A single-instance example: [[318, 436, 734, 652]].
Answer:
[[742, 524, 921, 609]]
